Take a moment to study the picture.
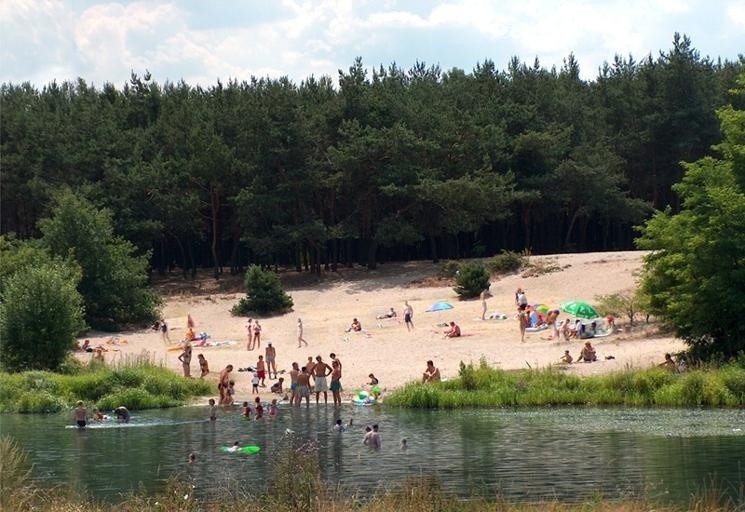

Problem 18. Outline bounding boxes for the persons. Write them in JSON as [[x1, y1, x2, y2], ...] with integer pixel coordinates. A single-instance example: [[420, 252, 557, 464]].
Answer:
[[158, 318, 171, 344], [296, 317, 309, 347], [344, 317, 362, 332], [333, 419, 346, 432], [93, 349, 104, 363], [513, 288, 616, 364], [82, 340, 107, 352], [72, 400, 89, 427], [176, 345, 192, 378], [402, 299, 414, 331], [366, 374, 379, 385], [187, 452, 197, 463], [346, 417, 353, 426], [230, 440, 240, 453], [186, 313, 194, 329], [361, 424, 382, 450], [365, 425, 373, 432], [111, 405, 130, 423], [421, 359, 441, 382], [479, 288, 489, 321], [399, 438, 408, 449], [443, 321, 461, 341], [92, 404, 102, 421], [195, 353, 210, 378], [375, 307, 398, 320], [208, 342, 343, 423], [245, 317, 252, 349], [251, 320, 262, 348]]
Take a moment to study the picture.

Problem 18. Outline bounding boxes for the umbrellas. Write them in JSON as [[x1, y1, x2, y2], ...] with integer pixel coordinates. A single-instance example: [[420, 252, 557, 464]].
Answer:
[[424, 303, 454, 315]]
